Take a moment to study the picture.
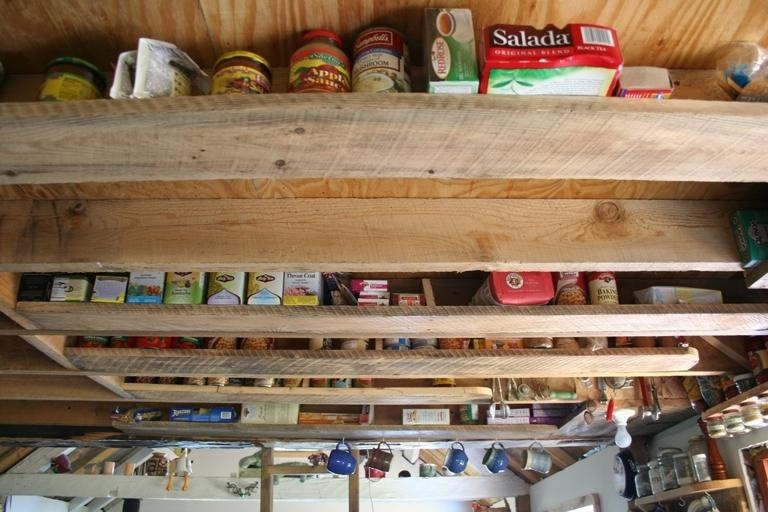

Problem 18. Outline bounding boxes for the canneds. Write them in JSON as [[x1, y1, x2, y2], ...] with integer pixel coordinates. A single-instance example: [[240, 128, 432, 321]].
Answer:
[[287, 31, 351, 93], [556, 271, 618, 307], [137, 374, 377, 387], [171, 60, 199, 96], [50, 269, 324, 306], [209, 50, 274, 95], [352, 24, 413, 94], [309, 336, 676, 352], [74, 333, 278, 349], [37, 56, 100, 103], [705, 373, 768, 438]]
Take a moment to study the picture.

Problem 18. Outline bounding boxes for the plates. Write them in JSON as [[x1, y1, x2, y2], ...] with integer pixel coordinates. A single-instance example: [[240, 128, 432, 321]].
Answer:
[[450, 9, 474, 43]]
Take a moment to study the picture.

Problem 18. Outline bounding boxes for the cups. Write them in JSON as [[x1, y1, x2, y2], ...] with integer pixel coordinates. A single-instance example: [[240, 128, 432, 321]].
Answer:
[[326, 441, 553, 478], [86, 461, 135, 475], [436, 9, 468, 36]]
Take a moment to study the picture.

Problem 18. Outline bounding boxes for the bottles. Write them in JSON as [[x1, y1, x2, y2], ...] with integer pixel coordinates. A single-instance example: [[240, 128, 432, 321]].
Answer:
[[146, 453, 168, 475], [470, 500, 480, 512], [634, 414, 727, 498], [682, 342, 767, 443]]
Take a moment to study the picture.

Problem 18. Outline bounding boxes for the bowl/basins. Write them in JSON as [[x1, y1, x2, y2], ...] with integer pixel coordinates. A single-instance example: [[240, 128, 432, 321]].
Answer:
[[611, 450, 636, 501]]
